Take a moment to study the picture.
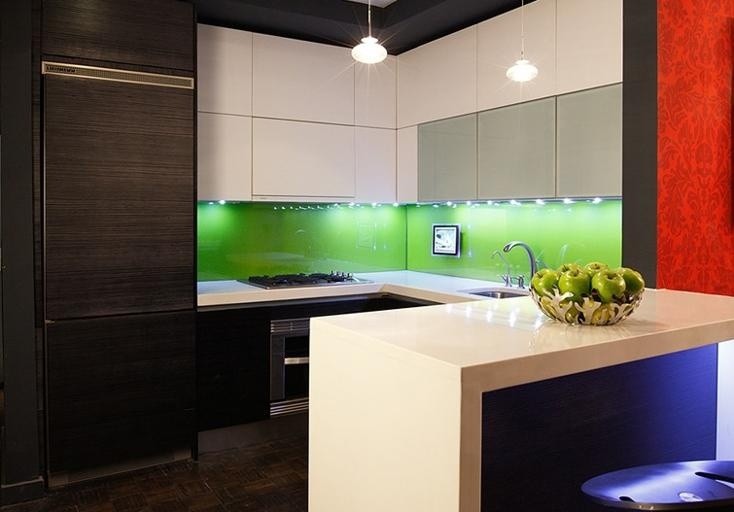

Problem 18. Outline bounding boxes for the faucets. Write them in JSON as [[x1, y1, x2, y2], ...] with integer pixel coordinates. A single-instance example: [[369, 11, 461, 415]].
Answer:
[[502, 241, 537, 282]]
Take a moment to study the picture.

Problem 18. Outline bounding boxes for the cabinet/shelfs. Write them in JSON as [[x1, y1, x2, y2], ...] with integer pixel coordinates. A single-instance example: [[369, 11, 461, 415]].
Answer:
[[34, 1, 197, 497], [197, 24, 399, 203], [396, 0, 622, 202]]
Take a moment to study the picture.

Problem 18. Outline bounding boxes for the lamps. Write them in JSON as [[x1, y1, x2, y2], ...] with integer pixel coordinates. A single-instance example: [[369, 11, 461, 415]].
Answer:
[[504, 0, 539, 82], [351, 0, 390, 65]]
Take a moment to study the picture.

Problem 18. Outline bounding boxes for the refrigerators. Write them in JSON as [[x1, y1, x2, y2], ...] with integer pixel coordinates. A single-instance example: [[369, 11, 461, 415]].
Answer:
[[16, 56, 205, 496]]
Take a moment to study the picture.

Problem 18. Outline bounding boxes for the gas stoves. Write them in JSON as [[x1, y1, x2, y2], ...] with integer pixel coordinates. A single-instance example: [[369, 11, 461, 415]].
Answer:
[[234, 271, 375, 291]]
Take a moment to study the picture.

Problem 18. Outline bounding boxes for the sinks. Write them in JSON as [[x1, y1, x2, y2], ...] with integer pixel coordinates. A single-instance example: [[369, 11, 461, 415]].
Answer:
[[466, 285, 530, 299]]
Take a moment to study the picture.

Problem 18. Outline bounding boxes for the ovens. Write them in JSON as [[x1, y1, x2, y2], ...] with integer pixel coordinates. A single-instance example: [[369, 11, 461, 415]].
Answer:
[[260, 316, 308, 419]]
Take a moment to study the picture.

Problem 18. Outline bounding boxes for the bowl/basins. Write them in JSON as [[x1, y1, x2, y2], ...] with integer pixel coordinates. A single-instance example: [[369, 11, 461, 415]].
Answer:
[[530, 290, 643, 326]]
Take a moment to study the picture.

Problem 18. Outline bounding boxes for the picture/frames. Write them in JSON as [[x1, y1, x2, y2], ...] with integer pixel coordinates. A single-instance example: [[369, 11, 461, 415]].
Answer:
[[430, 223, 461, 259]]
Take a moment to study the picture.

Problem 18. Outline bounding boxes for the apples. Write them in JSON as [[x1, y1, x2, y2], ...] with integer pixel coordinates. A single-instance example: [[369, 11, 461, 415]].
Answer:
[[531, 262, 645, 322]]
